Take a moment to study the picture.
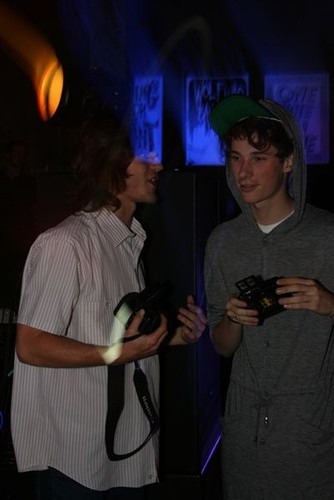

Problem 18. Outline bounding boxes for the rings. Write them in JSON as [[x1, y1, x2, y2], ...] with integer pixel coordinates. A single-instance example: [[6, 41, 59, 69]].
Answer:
[[234, 315, 239, 321]]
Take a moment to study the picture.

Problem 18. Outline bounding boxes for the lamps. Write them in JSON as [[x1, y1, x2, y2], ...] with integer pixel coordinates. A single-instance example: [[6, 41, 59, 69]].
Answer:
[[36, 58, 120, 130]]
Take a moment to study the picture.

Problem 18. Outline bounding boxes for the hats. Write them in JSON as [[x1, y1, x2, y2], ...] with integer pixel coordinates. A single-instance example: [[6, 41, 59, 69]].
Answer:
[[210, 95, 282, 139], [78, 112, 183, 163]]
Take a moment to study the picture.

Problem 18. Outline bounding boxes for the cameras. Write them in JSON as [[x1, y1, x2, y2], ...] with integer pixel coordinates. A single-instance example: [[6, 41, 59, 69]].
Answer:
[[235, 275, 292, 326], [112, 280, 185, 335]]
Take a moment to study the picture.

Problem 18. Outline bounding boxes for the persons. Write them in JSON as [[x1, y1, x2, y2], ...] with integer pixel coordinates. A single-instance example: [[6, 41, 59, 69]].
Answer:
[[11, 109, 205, 500], [203, 96, 334, 499]]
